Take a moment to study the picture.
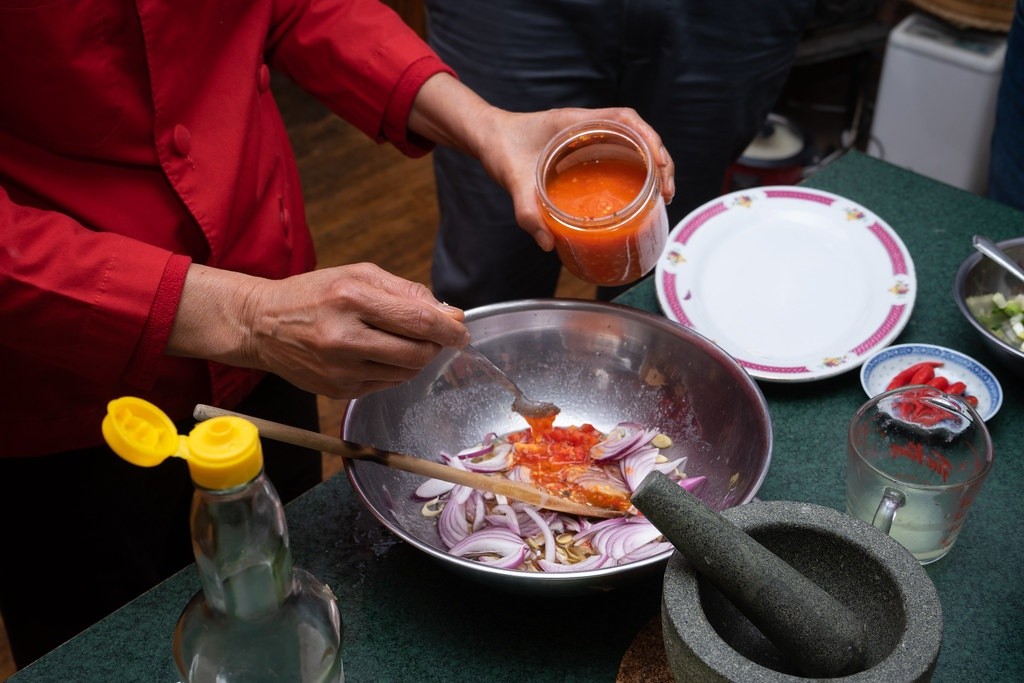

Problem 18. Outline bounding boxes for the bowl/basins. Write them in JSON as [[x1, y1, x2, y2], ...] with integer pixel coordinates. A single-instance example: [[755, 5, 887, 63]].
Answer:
[[953, 237, 1024, 378], [339, 297, 773, 580], [860, 342, 1003, 434], [661, 501, 942, 683]]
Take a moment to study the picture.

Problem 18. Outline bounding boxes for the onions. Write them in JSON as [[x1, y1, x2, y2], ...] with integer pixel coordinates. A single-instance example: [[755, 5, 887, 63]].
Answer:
[[412, 423, 705, 569]]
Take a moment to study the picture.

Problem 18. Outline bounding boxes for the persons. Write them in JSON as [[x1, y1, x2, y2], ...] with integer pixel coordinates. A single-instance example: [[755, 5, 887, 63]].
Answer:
[[422, 1, 798, 310], [0, 1, 674, 671]]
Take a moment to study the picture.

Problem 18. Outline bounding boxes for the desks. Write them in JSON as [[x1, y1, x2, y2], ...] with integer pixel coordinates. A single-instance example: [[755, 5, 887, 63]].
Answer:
[[0, 110, 1024, 682]]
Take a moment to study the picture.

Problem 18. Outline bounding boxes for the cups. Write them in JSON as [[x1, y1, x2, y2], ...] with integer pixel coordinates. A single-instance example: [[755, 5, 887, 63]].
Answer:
[[845, 385, 994, 566]]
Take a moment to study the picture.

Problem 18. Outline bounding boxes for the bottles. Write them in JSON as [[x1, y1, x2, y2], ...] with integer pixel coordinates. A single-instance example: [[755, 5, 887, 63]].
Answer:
[[538, 119, 668, 288], [103, 396, 346, 682]]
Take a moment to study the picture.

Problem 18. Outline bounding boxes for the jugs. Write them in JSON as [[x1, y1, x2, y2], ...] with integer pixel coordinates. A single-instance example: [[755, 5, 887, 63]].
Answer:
[[720, 111, 804, 194]]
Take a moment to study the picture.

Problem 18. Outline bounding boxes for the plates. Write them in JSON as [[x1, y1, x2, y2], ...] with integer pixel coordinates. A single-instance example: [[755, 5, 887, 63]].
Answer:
[[655, 184, 917, 383]]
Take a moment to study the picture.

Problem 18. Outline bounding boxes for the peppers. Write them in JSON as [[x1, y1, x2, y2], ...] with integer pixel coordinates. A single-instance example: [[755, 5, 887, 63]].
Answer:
[[887, 362, 974, 424]]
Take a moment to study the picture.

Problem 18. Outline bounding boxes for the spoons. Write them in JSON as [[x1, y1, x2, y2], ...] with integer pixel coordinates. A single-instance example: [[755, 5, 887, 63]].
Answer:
[[461, 343, 560, 417]]
